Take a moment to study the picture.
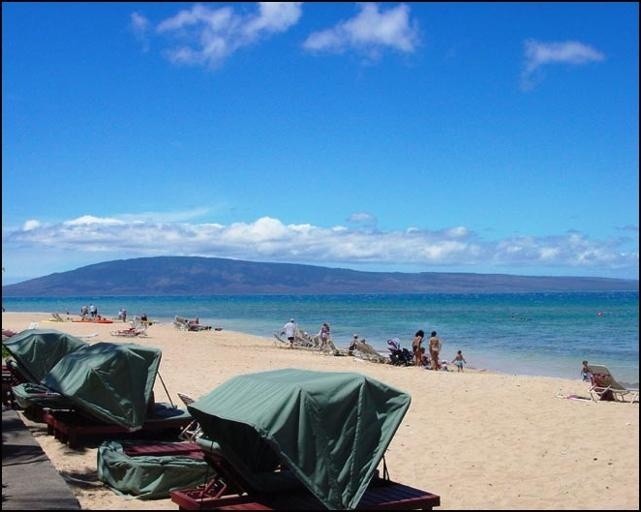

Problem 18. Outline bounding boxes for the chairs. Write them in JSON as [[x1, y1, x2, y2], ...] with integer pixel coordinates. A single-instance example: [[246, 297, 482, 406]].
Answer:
[[2, 366, 440, 511], [586, 366, 639, 403]]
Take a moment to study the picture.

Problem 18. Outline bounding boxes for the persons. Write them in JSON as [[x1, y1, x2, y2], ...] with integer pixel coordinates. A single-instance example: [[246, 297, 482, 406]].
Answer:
[[579, 359, 590, 381], [277, 319, 468, 372], [81, 303, 148, 325]]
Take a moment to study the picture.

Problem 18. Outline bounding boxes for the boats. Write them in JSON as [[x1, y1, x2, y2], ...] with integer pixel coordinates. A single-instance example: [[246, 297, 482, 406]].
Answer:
[[71, 315, 113, 323]]
